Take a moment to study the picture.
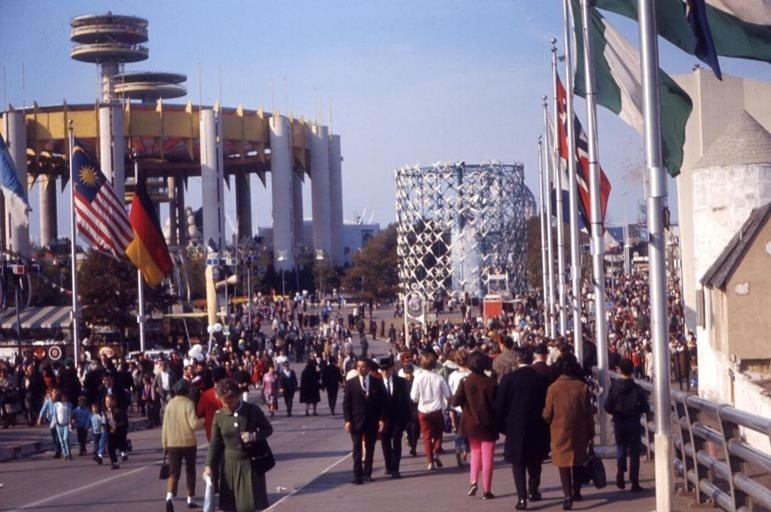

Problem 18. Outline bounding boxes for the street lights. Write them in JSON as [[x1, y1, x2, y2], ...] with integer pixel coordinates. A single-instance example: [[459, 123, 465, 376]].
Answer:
[[314, 248, 324, 292], [276, 248, 287, 296]]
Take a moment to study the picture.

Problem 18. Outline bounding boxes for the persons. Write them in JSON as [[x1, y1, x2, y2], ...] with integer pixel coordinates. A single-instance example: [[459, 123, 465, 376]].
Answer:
[[342, 272, 698, 510], [210, 288, 369, 418], [161, 368, 272, 512], [1, 346, 219, 470]]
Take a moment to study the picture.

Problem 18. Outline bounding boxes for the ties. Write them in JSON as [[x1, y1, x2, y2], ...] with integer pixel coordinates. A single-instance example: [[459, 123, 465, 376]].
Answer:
[[386, 378, 391, 394], [362, 376, 368, 395]]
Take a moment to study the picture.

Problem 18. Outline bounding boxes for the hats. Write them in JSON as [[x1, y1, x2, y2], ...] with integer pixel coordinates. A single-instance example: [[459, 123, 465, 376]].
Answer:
[[378, 357, 395, 369], [533, 342, 551, 354]]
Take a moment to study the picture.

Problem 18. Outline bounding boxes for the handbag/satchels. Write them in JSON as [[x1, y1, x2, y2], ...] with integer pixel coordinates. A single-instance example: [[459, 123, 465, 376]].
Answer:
[[581, 440, 607, 489], [247, 444, 276, 475], [158, 451, 171, 480]]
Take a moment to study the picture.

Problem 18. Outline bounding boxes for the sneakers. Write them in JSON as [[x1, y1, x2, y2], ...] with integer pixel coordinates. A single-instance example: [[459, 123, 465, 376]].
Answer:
[[427, 463, 436, 471], [165, 499, 176, 512], [351, 476, 364, 486], [574, 489, 584, 497], [483, 491, 495, 499], [615, 471, 625, 488], [384, 471, 401, 480], [563, 497, 574, 509], [631, 483, 643, 492], [186, 499, 202, 509], [515, 498, 529, 510], [467, 483, 478, 496], [364, 474, 379, 483], [433, 452, 443, 467], [529, 489, 543, 500]]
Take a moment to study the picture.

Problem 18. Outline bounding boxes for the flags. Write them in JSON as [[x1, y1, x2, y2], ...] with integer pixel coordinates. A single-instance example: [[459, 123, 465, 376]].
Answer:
[[72, 139, 136, 265], [550, 0, 770, 243], [124, 182, 174, 289], [0, 134, 34, 228]]
